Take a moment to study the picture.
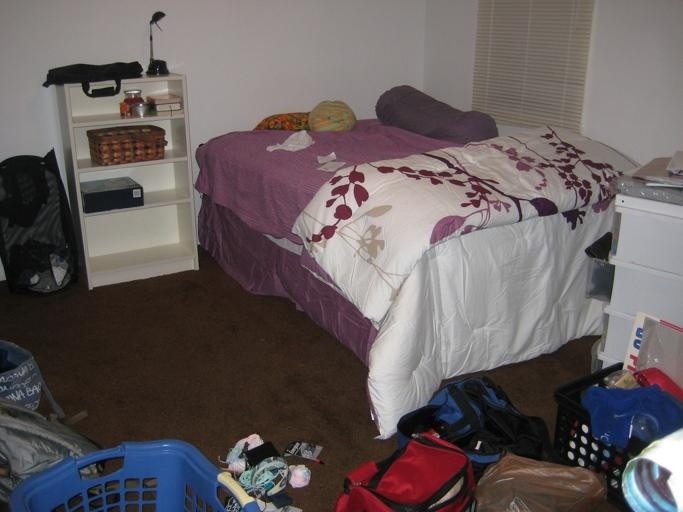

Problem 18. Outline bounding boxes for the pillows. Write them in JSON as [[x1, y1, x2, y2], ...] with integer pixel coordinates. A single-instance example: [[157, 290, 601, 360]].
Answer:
[[374, 84, 500, 143], [250, 109, 309, 136], [307, 99, 360, 132]]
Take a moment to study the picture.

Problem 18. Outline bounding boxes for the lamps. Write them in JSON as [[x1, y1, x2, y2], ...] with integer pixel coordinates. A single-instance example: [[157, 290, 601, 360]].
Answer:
[[145, 8, 174, 78]]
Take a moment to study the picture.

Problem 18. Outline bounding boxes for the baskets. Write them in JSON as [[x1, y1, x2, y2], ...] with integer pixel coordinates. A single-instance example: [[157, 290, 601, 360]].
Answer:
[[552, 359, 652, 512], [85, 124, 168, 167], [7, 438, 265, 512]]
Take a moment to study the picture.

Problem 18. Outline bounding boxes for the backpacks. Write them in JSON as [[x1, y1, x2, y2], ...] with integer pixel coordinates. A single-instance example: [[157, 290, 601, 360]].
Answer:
[[0, 394, 109, 512], [330, 430, 478, 512], [399, 373, 551, 483]]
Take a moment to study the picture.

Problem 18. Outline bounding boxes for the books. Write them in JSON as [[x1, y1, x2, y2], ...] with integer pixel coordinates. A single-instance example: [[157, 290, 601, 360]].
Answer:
[[147, 95, 183, 116]]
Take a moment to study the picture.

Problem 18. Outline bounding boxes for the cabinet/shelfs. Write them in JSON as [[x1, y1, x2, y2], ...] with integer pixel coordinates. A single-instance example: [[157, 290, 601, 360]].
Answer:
[[53, 70, 202, 292], [580, 162, 683, 377]]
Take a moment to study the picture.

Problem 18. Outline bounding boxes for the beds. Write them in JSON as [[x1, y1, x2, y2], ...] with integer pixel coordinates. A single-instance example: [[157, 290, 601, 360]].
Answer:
[[190, 106, 641, 444]]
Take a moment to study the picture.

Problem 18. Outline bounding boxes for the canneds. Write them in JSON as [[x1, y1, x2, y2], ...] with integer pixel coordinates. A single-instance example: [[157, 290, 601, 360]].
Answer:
[[124, 90, 144, 117], [131, 102, 151, 118]]
